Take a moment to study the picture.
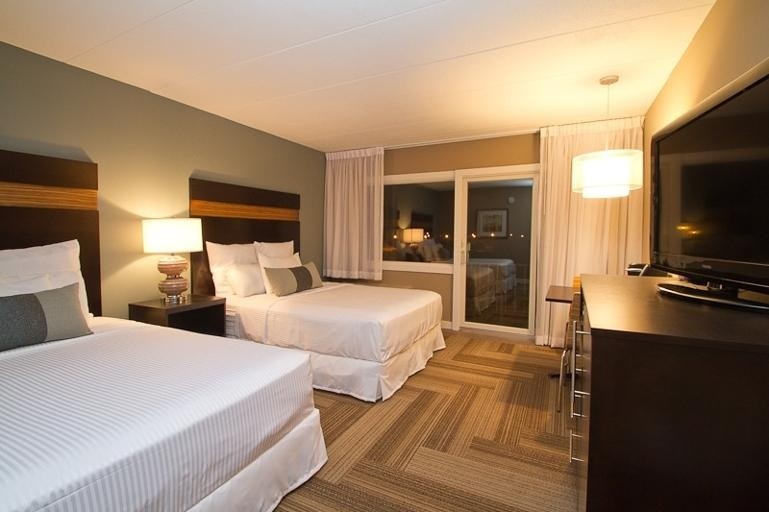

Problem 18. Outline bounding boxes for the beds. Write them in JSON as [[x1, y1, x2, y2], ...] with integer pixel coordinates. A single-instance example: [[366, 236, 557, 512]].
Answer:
[[383, 207, 498, 318], [0, 145, 331, 512], [410, 210, 519, 298], [187, 177, 447, 404]]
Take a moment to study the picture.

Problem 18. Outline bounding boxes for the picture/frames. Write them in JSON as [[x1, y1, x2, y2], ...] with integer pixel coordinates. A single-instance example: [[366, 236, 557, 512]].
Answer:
[[474, 208, 510, 239]]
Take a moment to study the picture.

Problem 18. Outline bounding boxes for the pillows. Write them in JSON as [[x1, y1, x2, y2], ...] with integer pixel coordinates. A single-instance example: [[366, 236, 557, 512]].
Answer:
[[205, 239, 259, 276], [0, 280, 95, 354], [224, 262, 266, 298], [253, 239, 295, 260], [210, 264, 236, 298], [256, 251, 302, 296], [262, 262, 324, 298], [415, 237, 451, 262], [0, 238, 94, 324]]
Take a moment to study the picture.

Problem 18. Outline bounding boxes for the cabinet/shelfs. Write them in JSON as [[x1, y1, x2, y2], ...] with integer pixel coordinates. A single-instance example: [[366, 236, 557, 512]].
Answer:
[[564, 273, 769, 510]]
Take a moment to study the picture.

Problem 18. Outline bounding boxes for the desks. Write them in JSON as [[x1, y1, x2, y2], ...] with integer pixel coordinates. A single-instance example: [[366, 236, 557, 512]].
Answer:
[[544, 283, 584, 381]]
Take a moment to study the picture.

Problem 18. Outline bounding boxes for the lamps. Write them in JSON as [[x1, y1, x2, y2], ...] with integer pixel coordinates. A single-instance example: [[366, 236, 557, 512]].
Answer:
[[140, 217, 203, 306], [568, 72, 645, 200], [403, 226, 425, 256]]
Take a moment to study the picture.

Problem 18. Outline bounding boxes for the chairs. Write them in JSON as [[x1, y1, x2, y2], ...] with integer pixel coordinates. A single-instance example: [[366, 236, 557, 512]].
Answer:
[[554, 291, 584, 412], [572, 274, 581, 289]]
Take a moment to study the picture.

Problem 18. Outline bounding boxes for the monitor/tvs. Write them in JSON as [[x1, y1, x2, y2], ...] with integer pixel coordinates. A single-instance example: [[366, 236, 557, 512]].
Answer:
[[650, 56, 769, 312]]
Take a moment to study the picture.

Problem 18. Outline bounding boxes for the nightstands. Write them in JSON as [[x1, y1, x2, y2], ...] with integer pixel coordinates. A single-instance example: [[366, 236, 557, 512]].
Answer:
[[407, 251, 422, 262], [127, 293, 228, 338]]
